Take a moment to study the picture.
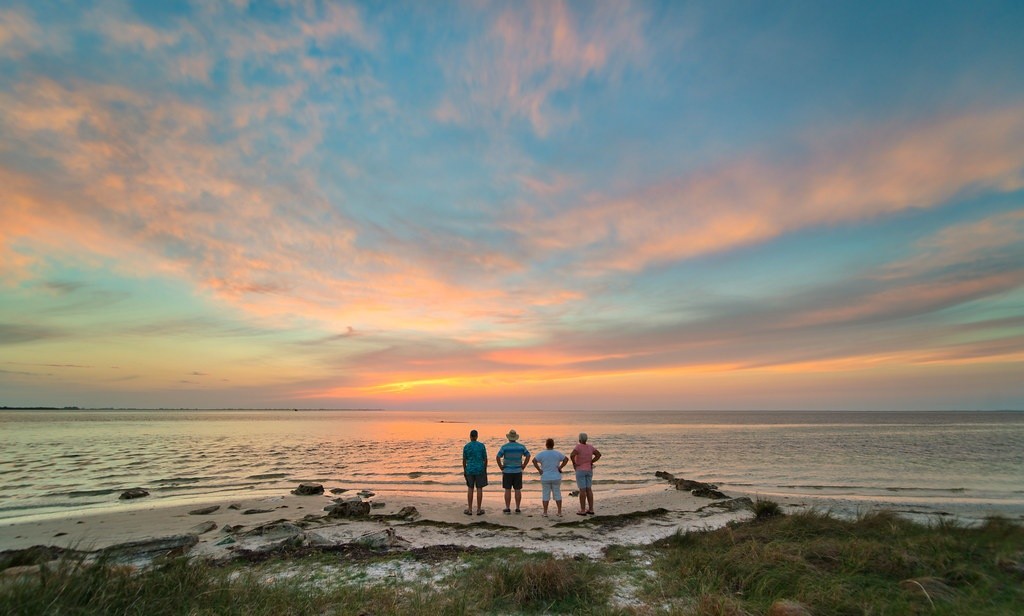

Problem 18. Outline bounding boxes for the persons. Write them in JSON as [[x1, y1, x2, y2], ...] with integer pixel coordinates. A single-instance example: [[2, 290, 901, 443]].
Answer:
[[532, 438, 569, 517], [462, 430, 488, 515], [570, 433, 602, 515], [496, 429, 530, 513]]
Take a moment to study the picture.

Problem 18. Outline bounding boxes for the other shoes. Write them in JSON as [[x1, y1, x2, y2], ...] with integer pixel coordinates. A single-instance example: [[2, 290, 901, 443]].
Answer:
[[515, 509, 521, 512], [586, 510, 594, 514], [503, 509, 510, 512], [556, 512, 563, 516], [477, 510, 485, 515], [577, 511, 586, 516], [464, 510, 472, 515], [542, 512, 548, 517]]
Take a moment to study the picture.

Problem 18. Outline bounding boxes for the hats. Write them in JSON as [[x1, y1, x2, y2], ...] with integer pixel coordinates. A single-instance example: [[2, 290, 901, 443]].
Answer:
[[506, 430, 519, 440], [471, 430, 477, 437]]
